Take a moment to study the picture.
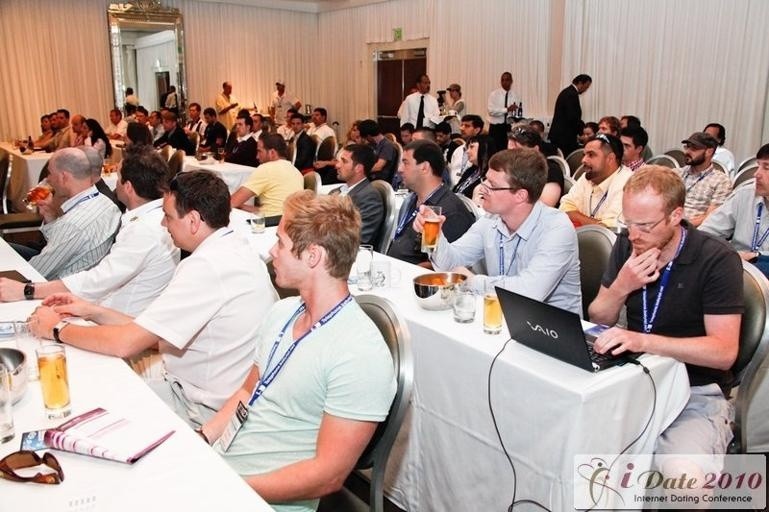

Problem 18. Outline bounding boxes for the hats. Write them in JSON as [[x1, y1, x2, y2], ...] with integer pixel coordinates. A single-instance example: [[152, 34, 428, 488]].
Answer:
[[447, 84, 461, 91], [276, 80, 285, 86], [682, 132, 718, 149]]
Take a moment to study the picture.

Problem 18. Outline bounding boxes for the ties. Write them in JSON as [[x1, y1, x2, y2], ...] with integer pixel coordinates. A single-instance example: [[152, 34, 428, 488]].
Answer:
[[416, 96, 425, 128], [505, 92, 508, 124]]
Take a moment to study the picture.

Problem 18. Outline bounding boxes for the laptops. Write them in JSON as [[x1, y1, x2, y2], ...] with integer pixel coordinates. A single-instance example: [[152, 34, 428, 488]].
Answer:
[[494, 287, 644, 373]]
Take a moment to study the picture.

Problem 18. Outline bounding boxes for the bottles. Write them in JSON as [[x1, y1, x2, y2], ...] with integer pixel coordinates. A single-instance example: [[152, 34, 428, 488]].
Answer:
[[512, 102, 522, 117], [27, 136, 34, 150]]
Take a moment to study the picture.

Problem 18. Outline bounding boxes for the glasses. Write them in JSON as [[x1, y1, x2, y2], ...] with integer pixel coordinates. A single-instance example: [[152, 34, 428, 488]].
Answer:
[[617, 211, 672, 234], [0, 449, 64, 483], [480, 177, 518, 192]]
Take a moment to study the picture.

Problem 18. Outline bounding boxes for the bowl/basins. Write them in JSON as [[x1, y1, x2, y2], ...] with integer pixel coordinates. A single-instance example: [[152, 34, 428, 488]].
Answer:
[[412, 272, 467, 311]]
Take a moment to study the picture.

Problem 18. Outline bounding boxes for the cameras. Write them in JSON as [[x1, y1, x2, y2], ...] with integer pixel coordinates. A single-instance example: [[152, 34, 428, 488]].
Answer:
[[437, 90, 446, 106]]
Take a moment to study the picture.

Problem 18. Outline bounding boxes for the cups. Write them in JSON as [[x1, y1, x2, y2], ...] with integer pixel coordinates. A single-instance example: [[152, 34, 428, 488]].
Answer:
[[0, 364, 15, 445], [451, 288, 476, 322], [483, 294, 503, 334], [35, 344, 72, 420], [356, 244, 373, 292], [19, 144, 26, 154], [250, 213, 265, 234], [421, 206, 442, 248], [21, 177, 53, 211], [218, 148, 224, 164]]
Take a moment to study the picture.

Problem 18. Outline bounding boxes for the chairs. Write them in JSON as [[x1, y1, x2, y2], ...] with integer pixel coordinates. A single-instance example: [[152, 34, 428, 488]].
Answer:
[[0, 115, 769, 512]]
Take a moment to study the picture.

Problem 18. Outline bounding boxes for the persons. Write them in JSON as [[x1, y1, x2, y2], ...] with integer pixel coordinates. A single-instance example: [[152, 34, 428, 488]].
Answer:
[[0, 72, 767, 511]]
